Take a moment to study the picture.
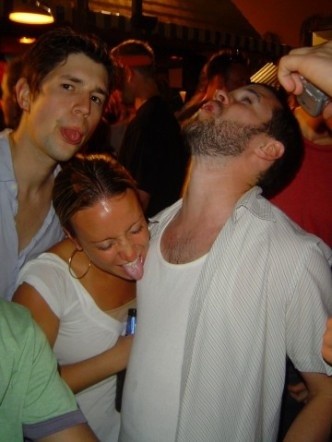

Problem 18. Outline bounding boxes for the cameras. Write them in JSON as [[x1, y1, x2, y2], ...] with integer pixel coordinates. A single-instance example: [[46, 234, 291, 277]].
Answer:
[[297, 76, 330, 117]]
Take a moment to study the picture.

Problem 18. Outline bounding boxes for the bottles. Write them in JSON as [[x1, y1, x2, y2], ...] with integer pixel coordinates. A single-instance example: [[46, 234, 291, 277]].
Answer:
[[115, 309, 137, 413]]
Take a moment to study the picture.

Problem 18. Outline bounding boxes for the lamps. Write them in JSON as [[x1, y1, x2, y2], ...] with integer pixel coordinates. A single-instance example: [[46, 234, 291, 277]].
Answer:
[[10, 0, 54, 26]]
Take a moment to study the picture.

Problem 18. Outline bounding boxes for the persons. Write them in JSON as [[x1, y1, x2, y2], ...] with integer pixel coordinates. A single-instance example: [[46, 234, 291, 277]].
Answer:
[[269, 39, 332, 367], [12, 158, 150, 442], [109, 38, 192, 222], [0, 299, 100, 442], [0, 31, 118, 302], [118, 83, 332, 442], [172, 51, 245, 129]]
